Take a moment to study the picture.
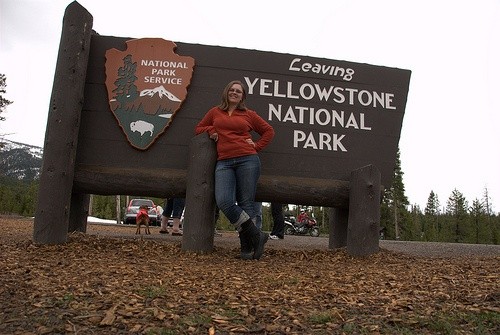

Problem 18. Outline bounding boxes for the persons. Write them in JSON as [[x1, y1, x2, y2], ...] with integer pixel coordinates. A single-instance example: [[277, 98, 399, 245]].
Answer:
[[159, 197, 186, 236], [214, 202, 285, 240], [194, 81, 275, 260], [298, 209, 308, 224], [285, 207, 291, 215], [156, 202, 163, 227]]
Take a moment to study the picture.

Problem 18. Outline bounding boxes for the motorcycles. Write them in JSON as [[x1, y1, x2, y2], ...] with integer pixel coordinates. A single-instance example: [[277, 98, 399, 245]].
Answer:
[[285, 212, 320, 237]]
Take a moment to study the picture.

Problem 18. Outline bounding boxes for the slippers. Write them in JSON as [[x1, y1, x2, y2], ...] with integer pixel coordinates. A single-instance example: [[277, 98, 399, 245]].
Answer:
[[172, 230, 183, 236], [159, 229, 169, 233]]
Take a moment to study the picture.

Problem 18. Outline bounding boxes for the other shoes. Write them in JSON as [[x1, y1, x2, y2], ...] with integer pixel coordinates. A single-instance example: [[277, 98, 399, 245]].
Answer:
[[268, 235, 284, 240]]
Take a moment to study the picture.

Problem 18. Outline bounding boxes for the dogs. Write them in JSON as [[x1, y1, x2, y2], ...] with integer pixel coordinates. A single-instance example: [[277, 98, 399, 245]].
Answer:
[[135, 205, 151, 236]]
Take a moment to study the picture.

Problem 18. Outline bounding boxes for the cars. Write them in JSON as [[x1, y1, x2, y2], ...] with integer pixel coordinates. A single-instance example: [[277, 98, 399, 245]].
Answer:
[[122, 198, 158, 226], [156, 204, 186, 229]]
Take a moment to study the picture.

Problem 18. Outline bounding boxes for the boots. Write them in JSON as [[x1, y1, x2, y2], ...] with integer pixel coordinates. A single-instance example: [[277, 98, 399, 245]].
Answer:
[[238, 231, 254, 259], [240, 218, 269, 260]]
